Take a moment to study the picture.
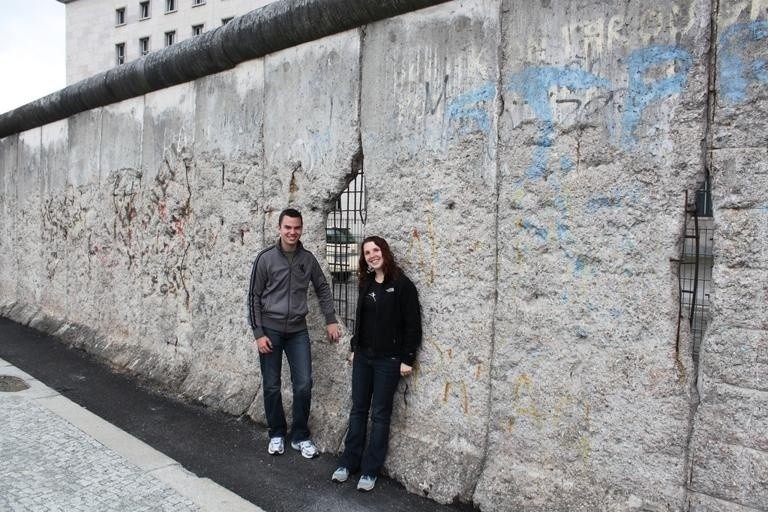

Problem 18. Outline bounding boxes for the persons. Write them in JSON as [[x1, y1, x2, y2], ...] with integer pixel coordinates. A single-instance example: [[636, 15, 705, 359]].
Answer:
[[245, 207, 343, 459], [330, 233, 422, 494]]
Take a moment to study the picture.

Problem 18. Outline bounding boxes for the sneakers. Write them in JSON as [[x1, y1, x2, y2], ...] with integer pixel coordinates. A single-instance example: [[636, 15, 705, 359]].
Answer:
[[332, 467, 349, 483], [357, 474, 378, 491], [291, 439, 320, 459], [268, 437, 286, 455]]
